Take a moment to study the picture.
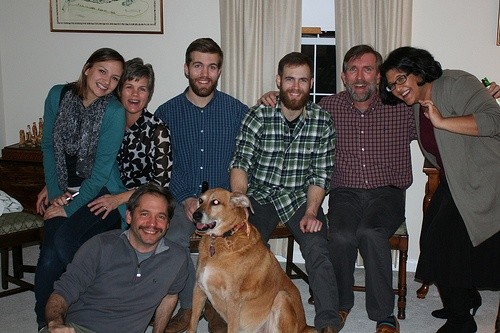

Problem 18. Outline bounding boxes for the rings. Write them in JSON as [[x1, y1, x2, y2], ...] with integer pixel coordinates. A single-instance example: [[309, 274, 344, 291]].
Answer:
[[104, 206, 107, 209], [432, 105, 435, 107]]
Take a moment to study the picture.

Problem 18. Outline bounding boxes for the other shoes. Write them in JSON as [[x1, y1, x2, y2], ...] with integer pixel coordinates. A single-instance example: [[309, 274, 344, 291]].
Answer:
[[202, 302, 227, 333], [164, 307, 204, 333], [376, 315, 400, 333], [338, 310, 350, 332]]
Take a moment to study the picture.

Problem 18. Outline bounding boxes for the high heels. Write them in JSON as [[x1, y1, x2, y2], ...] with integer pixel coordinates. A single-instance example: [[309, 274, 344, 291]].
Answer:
[[435, 311, 477, 333], [432, 288, 481, 319]]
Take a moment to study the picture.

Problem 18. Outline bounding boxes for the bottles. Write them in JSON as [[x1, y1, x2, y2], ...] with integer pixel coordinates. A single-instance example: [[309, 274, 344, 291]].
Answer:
[[27, 125, 32, 141], [39, 118, 44, 135], [32, 122, 37, 137]]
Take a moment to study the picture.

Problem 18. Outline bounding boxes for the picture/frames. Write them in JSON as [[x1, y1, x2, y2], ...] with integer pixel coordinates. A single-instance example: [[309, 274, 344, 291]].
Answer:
[[50, 0, 164, 35]]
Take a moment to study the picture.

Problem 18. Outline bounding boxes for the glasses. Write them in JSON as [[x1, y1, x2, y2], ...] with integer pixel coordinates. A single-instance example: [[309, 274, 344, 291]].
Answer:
[[385, 71, 413, 93]]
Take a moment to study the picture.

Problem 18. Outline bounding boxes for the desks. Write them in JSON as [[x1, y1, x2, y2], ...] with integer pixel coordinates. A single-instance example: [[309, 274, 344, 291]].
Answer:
[[0, 142, 43, 207]]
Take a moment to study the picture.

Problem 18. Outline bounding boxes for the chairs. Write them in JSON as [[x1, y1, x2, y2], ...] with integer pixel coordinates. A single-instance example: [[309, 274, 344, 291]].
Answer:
[[0, 207, 46, 298], [270, 220, 315, 305], [353, 219, 409, 320]]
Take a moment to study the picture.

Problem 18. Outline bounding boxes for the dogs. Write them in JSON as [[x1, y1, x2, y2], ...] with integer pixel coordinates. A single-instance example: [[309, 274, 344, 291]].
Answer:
[[182, 187, 318, 333]]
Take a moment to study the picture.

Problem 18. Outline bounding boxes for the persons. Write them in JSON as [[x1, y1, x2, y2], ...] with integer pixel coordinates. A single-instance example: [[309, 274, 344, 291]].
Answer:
[[228, 52, 340, 333], [257, 45, 500, 333], [46, 184, 190, 333], [36, 58, 175, 326], [379, 46, 500, 333], [152, 38, 249, 333], [34, 48, 128, 333]]
